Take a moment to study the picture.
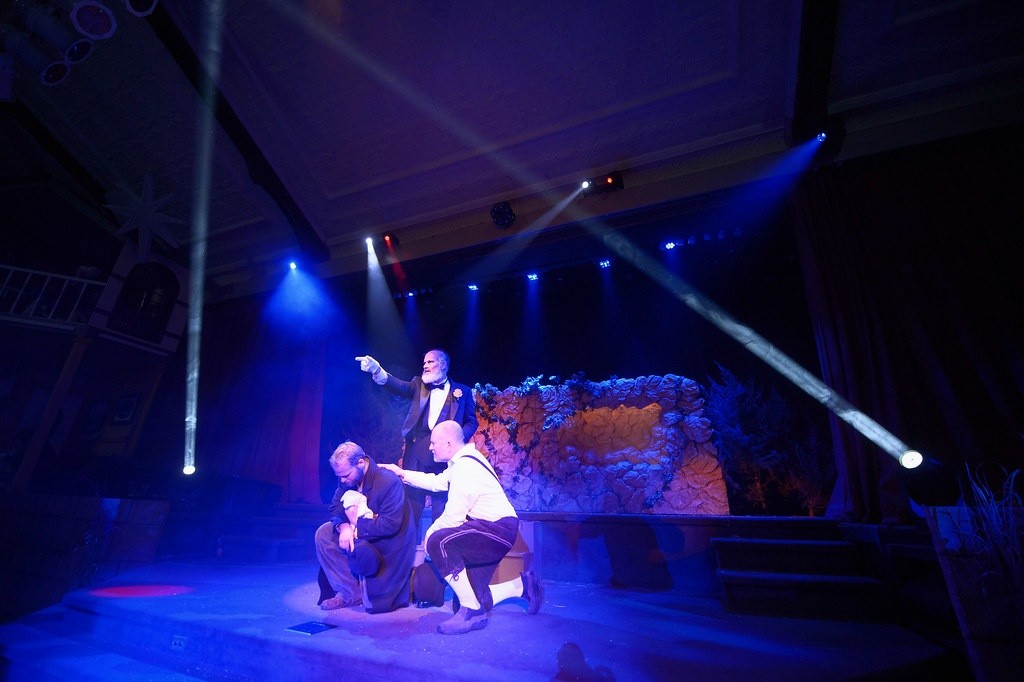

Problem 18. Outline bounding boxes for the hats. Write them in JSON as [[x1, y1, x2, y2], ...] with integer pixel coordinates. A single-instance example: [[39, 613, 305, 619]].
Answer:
[[412, 564, 444, 608], [347, 541, 381, 577]]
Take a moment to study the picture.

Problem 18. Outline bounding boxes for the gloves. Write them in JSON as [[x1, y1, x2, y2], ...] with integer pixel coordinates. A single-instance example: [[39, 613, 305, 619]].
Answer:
[[355, 356, 379, 373]]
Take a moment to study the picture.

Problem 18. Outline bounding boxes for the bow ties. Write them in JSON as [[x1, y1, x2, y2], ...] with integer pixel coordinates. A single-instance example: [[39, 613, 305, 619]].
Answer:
[[424, 384, 444, 391]]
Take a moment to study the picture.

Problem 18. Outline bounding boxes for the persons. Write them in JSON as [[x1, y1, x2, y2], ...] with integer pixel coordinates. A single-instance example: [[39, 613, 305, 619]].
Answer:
[[314, 439, 417, 615], [376, 419, 544, 636], [355, 349, 479, 544]]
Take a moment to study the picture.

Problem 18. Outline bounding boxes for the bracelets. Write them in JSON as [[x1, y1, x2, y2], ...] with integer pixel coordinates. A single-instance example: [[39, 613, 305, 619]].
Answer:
[[374, 366, 381, 375]]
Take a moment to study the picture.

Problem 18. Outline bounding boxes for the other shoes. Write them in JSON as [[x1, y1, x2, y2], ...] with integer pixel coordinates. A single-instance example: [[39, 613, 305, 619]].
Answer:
[[322, 597, 365, 610], [521, 569, 544, 614], [437, 605, 487, 634]]
